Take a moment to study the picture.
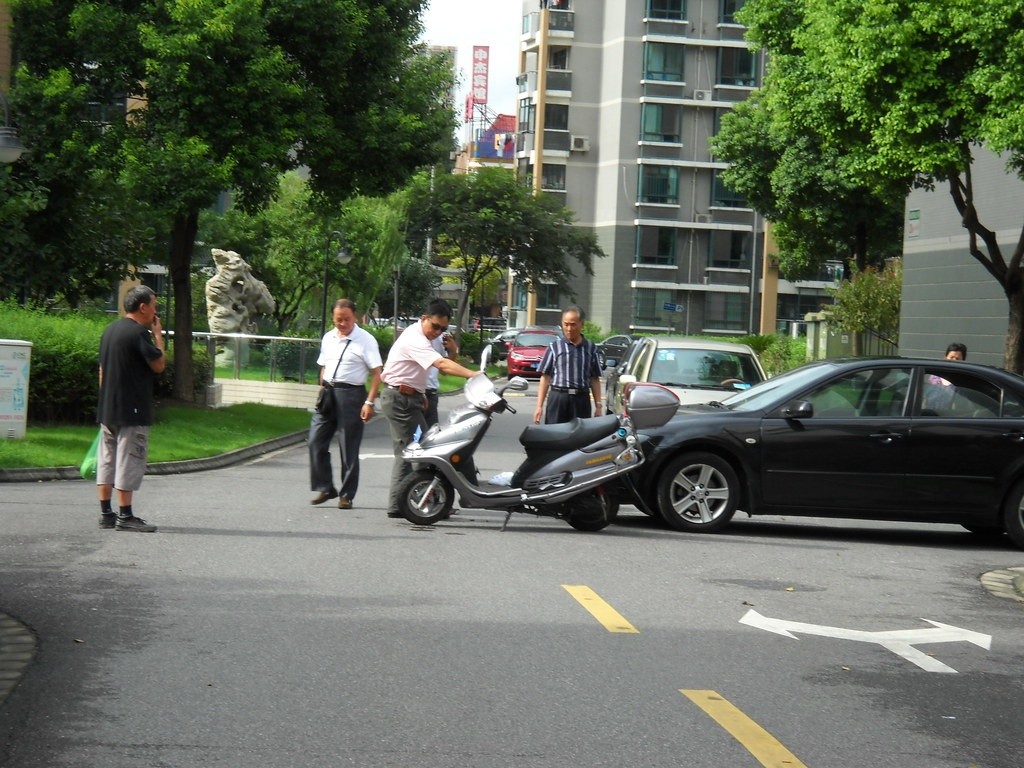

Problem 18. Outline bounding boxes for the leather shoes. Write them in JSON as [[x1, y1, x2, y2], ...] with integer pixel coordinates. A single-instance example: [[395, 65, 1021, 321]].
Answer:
[[339, 496, 352, 508], [311, 488, 338, 505]]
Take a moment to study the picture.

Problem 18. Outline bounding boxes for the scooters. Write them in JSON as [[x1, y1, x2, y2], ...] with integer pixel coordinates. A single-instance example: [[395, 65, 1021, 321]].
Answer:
[[395, 381, 681, 534]]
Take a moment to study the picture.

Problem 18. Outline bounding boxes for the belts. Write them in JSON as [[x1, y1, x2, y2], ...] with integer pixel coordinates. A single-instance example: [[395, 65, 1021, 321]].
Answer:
[[551, 386, 589, 394], [323, 381, 363, 387], [426, 389, 437, 395], [385, 383, 421, 396]]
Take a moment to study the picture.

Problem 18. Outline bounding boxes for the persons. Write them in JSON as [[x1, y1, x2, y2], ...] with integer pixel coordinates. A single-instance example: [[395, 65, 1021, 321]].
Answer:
[[922, 343, 967, 410], [381, 298, 481, 518], [534, 305, 603, 425], [423, 334, 457, 428], [97, 285, 165, 531], [308, 299, 383, 509]]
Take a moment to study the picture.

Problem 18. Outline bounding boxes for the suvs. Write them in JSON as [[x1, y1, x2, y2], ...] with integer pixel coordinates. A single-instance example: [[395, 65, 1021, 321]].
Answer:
[[473, 317, 507, 333]]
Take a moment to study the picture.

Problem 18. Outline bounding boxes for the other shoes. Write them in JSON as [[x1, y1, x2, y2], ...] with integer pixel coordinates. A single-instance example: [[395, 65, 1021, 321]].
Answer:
[[388, 512, 404, 518]]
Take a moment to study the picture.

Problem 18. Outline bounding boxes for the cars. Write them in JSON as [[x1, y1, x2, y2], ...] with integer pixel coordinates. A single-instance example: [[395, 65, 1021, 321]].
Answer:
[[446, 324, 466, 339], [605, 337, 769, 420], [624, 353, 1024, 551], [368, 315, 420, 333], [593, 333, 645, 371], [488, 329, 528, 361], [504, 330, 562, 382]]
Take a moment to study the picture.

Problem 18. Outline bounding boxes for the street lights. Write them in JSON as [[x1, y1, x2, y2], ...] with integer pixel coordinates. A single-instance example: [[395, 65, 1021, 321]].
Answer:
[[319, 229, 353, 338]]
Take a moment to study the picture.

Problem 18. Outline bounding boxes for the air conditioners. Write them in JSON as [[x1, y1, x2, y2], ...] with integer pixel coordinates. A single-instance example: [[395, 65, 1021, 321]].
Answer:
[[694, 90, 712, 100], [570, 135, 590, 151], [695, 214, 713, 223]]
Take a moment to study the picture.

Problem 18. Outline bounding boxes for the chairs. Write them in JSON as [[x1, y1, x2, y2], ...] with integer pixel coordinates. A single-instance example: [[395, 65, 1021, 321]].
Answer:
[[857, 383, 1019, 418], [652, 355, 742, 385]]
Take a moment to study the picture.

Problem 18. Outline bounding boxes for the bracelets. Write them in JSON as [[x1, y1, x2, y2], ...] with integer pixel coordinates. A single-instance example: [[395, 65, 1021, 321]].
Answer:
[[365, 401, 375, 406], [595, 402, 602, 407]]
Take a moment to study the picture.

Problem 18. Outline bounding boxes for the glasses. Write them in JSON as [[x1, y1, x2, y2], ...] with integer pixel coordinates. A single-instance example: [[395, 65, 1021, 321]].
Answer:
[[427, 317, 447, 332]]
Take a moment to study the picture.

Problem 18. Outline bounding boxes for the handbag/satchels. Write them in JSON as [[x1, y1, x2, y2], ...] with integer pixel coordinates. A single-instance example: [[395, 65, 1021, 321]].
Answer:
[[80, 429, 101, 480], [316, 388, 332, 413]]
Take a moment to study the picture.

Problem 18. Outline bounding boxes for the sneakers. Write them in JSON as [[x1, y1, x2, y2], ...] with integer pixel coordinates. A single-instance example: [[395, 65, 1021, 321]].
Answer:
[[115, 515, 158, 532], [98, 512, 116, 528]]
